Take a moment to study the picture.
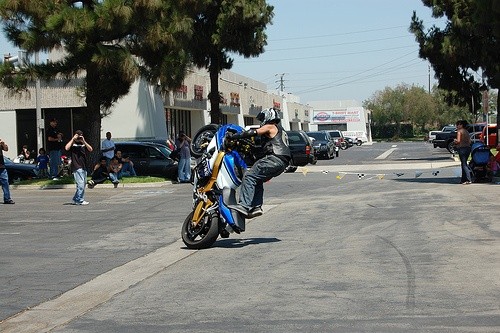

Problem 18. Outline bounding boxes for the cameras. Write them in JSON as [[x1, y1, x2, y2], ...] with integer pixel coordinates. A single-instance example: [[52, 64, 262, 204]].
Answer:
[[77, 135, 80, 139]]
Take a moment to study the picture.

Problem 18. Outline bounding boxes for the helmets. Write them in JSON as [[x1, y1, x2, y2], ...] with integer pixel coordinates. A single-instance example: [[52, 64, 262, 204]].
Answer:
[[256, 107, 280, 127]]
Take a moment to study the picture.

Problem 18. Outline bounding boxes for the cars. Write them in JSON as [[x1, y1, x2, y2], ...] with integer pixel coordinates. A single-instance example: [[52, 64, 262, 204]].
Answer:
[[3, 155, 40, 185], [114, 139, 196, 184], [428, 122, 500, 165]]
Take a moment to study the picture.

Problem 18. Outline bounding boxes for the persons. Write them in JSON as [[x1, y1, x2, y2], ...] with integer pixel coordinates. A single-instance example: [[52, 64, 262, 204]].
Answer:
[[65, 130, 93, 205], [101, 132, 116, 167], [0, 139, 16, 204], [88, 149, 136, 186], [453, 119, 473, 184], [227, 108, 291, 219], [21, 145, 49, 177], [176, 130, 191, 183], [46, 117, 64, 180], [47, 154, 51, 161]]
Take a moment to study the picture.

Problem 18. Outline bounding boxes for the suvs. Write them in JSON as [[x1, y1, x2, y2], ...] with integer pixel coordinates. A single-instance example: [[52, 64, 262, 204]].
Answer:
[[305, 130, 337, 160], [322, 130, 344, 150], [250, 129, 318, 174]]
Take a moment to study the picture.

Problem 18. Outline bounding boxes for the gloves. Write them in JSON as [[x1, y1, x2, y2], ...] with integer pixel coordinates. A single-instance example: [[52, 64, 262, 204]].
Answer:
[[232, 134, 241, 140]]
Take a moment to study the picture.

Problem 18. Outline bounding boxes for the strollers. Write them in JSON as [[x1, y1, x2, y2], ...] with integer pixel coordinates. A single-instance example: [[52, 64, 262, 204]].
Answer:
[[468, 141, 493, 183]]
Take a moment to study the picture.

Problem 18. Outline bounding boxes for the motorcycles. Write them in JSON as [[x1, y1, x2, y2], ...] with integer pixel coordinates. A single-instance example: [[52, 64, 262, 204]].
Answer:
[[180, 123, 255, 249]]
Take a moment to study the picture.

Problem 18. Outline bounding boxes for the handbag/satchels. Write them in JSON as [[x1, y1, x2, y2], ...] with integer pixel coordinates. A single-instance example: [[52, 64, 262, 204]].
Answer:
[[459, 132, 469, 144]]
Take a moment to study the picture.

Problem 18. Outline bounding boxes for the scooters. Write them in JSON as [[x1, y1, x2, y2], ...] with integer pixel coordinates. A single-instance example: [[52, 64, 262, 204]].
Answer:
[[343, 135, 362, 147]]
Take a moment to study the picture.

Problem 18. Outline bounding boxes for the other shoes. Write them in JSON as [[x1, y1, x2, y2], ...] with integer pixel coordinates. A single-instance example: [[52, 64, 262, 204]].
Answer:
[[466, 181, 472, 183], [113, 180, 119, 183], [4, 200, 15, 204], [459, 181, 466, 184], [247, 207, 262, 219], [180, 180, 191, 183], [88, 180, 94, 186], [227, 204, 248, 215], [72, 198, 89, 205]]
